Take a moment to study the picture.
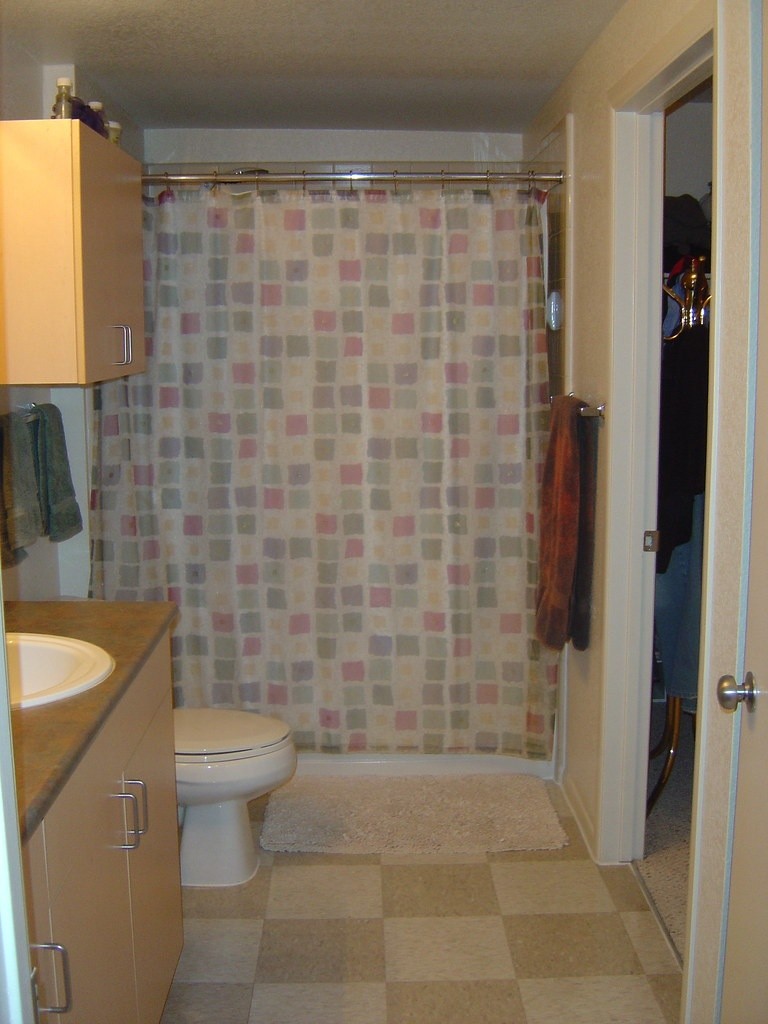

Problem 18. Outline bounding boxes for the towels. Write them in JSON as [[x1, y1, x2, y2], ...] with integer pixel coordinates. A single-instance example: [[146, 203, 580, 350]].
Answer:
[[28, 402, 84, 544], [0, 410, 43, 571], [532, 396, 599, 652]]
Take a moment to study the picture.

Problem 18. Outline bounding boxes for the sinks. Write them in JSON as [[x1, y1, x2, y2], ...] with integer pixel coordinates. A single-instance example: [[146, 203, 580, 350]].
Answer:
[[2, 632, 117, 711]]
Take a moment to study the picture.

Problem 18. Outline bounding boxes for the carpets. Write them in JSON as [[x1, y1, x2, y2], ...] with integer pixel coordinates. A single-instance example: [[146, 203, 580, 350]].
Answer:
[[259, 774, 570, 855]]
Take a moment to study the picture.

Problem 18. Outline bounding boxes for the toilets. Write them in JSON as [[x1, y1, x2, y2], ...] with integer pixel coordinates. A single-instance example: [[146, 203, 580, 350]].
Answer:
[[37, 594, 298, 888]]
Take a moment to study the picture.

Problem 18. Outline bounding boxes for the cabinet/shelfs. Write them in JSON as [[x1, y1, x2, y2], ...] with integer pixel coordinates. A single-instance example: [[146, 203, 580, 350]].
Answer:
[[0, 117, 151, 387], [23, 629, 184, 1024]]
